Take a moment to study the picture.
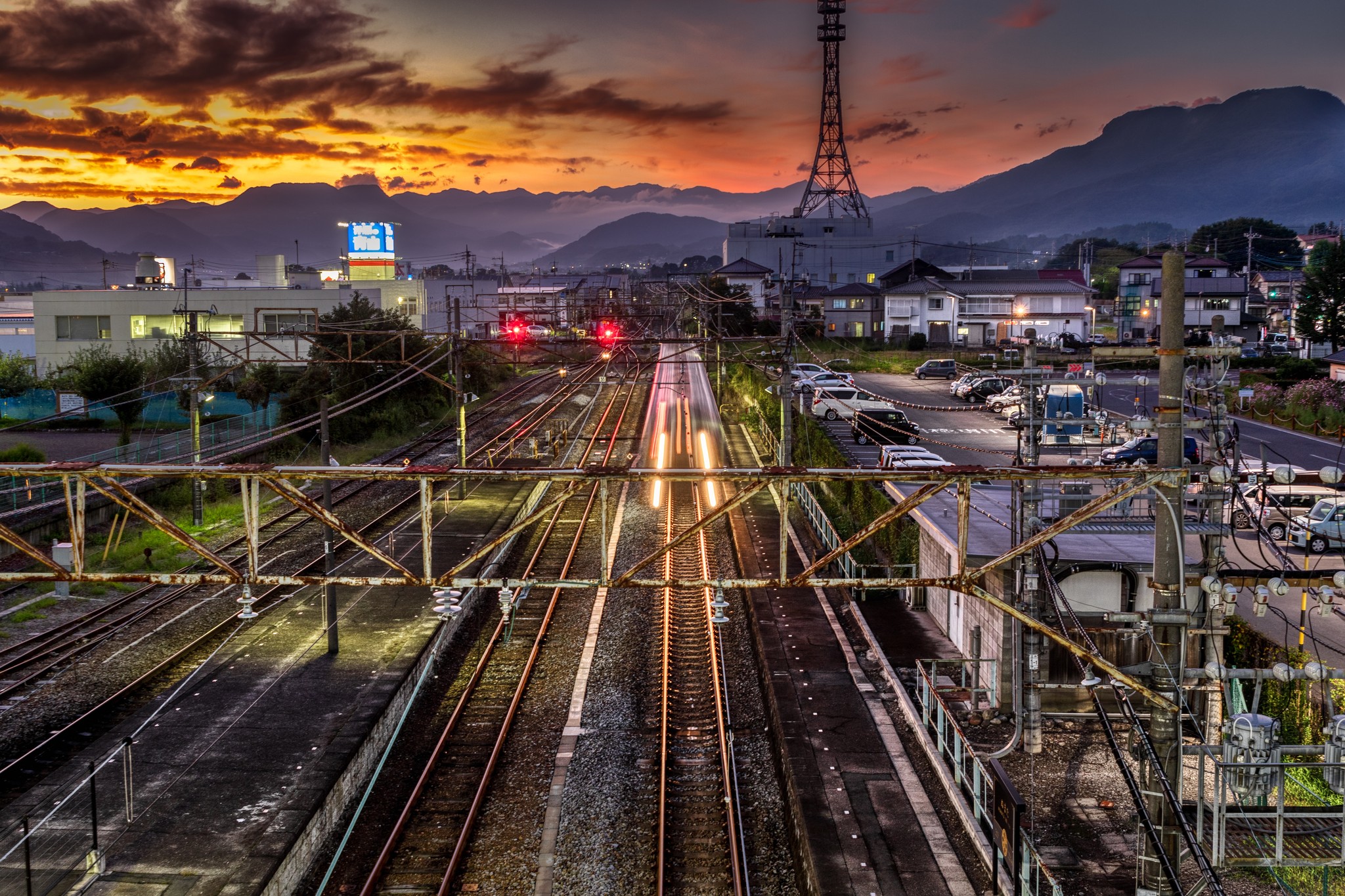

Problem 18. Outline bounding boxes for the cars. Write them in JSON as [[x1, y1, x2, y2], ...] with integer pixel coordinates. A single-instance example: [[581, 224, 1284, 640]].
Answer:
[[1185, 459, 1345, 529], [1239, 347, 1259, 360], [823, 360, 848, 367], [951, 373, 1090, 430], [1086, 334, 1108, 344], [498, 326, 511, 336]]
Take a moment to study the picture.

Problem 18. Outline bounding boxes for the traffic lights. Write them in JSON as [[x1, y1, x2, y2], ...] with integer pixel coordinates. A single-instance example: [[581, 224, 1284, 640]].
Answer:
[[1270, 292, 1282, 297], [604, 320, 614, 338], [514, 325, 520, 336]]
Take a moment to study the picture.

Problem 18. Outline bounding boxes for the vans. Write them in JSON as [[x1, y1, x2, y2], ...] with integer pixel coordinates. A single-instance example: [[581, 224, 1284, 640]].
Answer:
[[525, 326, 550, 337], [1250, 485, 1344, 540], [1263, 333, 1287, 348]]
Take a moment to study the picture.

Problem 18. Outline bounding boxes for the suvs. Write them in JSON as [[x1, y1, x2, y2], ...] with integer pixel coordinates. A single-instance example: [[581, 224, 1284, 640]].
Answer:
[[1285, 497, 1345, 554], [1098, 435, 1200, 468], [875, 445, 956, 469], [1262, 344, 1291, 358], [785, 361, 920, 444], [914, 359, 956, 380], [977, 339, 1021, 363]]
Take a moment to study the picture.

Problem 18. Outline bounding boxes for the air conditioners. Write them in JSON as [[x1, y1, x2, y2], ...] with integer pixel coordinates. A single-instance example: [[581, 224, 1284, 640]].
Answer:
[[985, 339, 995, 344], [1123, 331, 1132, 338], [294, 283, 306, 290]]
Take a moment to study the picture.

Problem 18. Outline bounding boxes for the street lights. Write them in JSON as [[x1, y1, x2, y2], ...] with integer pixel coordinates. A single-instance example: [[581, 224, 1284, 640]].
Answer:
[[1198, 291, 1204, 331], [946, 294, 954, 361], [189, 391, 214, 529], [1003, 299, 1014, 369], [1084, 304, 1096, 379]]
